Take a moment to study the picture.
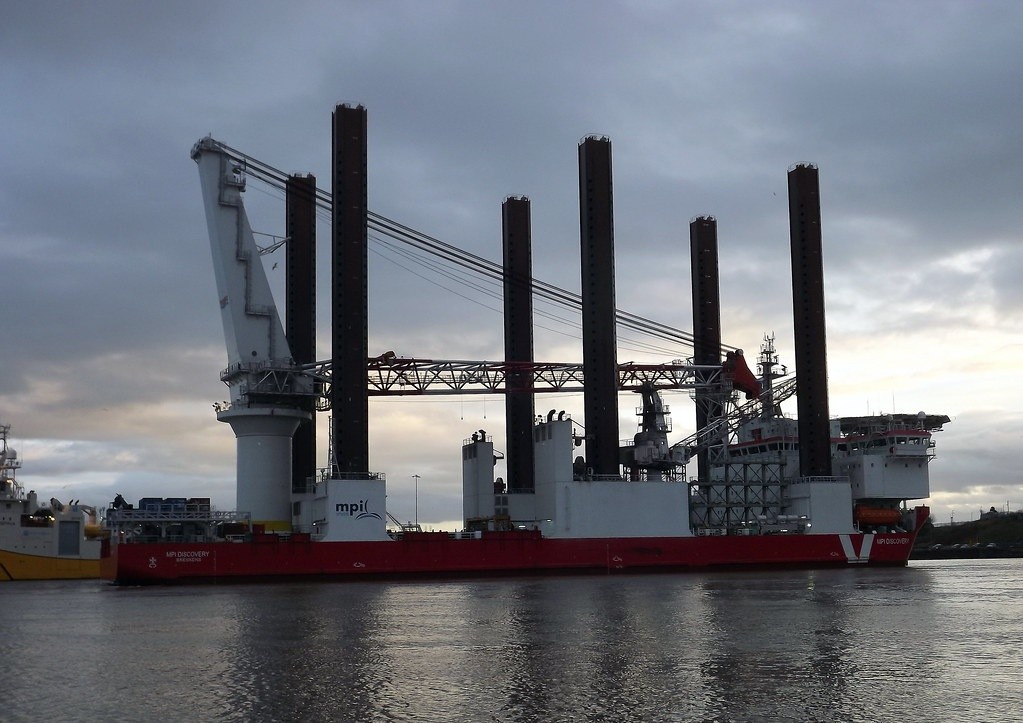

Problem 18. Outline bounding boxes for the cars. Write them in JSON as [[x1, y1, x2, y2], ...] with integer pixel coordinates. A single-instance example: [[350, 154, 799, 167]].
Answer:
[[932, 543, 997, 549]]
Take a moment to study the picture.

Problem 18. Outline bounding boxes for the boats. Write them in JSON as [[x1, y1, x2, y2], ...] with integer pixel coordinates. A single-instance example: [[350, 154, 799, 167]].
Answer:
[[0, 423, 111, 580], [110, 105, 952, 581]]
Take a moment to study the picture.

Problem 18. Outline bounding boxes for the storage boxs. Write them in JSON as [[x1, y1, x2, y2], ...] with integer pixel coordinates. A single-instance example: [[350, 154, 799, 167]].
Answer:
[[139, 498, 210, 519], [218, 523, 244, 537]]
